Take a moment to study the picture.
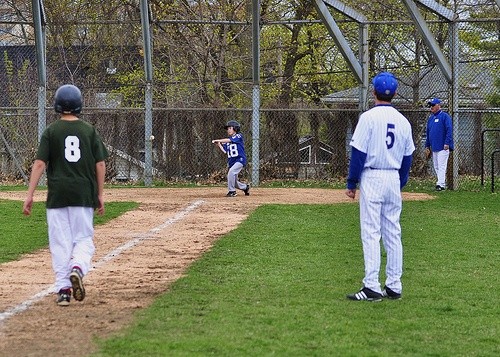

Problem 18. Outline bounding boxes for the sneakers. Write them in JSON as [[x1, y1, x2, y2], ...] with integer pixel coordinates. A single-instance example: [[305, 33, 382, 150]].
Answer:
[[243, 185, 250, 195], [346, 287, 382, 302], [382, 285, 402, 300], [69, 268, 86, 301], [56, 294, 70, 306], [225, 191, 237, 197]]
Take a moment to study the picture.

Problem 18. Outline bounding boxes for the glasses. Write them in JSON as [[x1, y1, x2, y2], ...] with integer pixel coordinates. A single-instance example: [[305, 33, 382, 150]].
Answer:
[[428, 104, 435, 107]]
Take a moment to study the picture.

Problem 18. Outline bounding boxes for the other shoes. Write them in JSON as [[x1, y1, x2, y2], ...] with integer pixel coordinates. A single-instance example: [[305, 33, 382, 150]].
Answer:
[[435, 186, 446, 192]]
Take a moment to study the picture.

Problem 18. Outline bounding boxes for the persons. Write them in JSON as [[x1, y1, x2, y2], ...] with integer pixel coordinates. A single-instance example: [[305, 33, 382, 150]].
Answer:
[[23, 84, 109, 306], [212, 120, 251, 197], [424, 98, 454, 192], [345, 72, 415, 302]]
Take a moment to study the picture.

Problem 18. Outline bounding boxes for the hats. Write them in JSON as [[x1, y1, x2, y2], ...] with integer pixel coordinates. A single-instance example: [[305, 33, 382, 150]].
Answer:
[[427, 97, 440, 104], [372, 72, 398, 97]]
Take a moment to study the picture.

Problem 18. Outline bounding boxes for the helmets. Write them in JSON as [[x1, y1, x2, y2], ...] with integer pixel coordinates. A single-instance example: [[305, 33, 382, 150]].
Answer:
[[223, 120, 240, 132], [53, 84, 82, 114]]
